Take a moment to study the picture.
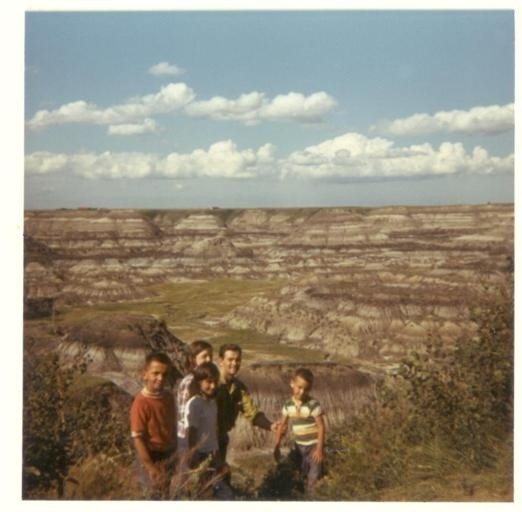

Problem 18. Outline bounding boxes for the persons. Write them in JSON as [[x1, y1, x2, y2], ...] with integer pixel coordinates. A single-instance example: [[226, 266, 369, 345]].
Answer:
[[181, 362, 223, 501], [211, 344, 288, 500], [126, 353, 177, 500], [272, 366, 326, 501], [165, 339, 213, 501]]
